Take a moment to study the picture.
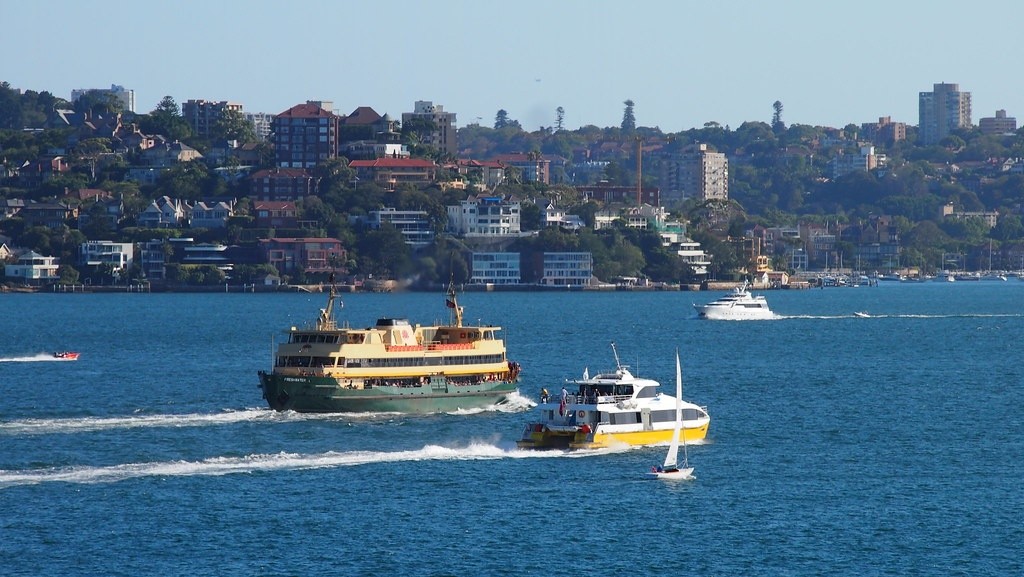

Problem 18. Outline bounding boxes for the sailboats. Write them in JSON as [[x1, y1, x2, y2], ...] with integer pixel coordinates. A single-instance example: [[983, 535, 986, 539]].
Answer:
[[642, 348, 698, 480]]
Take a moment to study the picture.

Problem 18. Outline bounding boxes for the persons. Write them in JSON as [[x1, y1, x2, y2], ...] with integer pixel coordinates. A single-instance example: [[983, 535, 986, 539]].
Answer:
[[344, 385, 358, 389], [593, 388, 618, 399], [582, 422, 593, 435], [571, 391, 581, 396], [282, 356, 334, 377], [363, 359, 522, 389], [652, 464, 663, 473], [559, 386, 569, 404], [541, 388, 549, 403]]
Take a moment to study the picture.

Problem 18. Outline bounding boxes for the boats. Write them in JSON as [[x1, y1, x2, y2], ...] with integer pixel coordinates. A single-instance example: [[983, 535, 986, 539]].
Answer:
[[854, 311, 870, 317], [819, 266, 1024, 290], [54, 352, 81, 361], [691, 279, 776, 320], [255, 270, 522, 414], [514, 342, 710, 455]]
